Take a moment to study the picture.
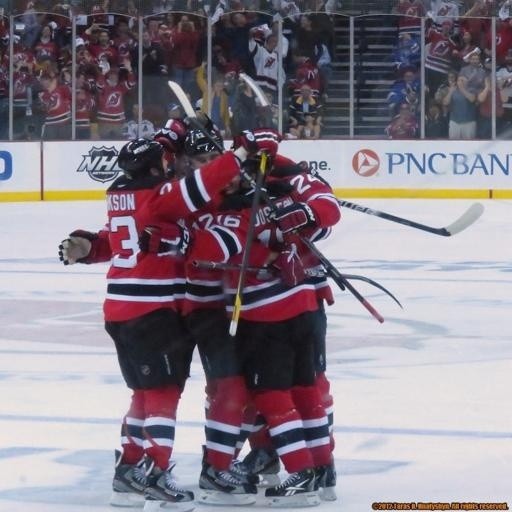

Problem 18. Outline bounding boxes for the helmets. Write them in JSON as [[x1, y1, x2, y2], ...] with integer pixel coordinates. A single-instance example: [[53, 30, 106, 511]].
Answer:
[[183, 124, 225, 155], [6, 45, 120, 90], [117, 137, 165, 181]]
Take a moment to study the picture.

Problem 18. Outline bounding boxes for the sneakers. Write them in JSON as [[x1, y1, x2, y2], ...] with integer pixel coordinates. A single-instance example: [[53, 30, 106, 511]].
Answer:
[[111, 445, 338, 501]]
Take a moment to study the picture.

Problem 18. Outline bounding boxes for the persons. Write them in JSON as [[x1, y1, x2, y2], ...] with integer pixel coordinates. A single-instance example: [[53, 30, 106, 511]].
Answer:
[[58, 120, 258, 494], [137, 162, 337, 497], [153, 119, 259, 484], [231, 128, 342, 475], [103, 127, 284, 502], [1, 0, 336, 140], [385, 1, 512, 142]]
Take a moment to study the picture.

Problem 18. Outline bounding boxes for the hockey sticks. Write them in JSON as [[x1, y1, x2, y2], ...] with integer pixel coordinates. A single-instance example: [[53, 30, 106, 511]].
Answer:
[[192, 260, 403, 311], [168, 80, 384, 324], [229, 69, 271, 337], [339, 200, 485, 236]]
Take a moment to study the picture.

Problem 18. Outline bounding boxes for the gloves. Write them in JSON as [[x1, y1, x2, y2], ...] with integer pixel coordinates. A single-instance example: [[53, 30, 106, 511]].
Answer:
[[268, 201, 315, 235], [137, 223, 191, 259], [232, 126, 279, 158], [156, 119, 188, 154], [58, 229, 98, 265]]
